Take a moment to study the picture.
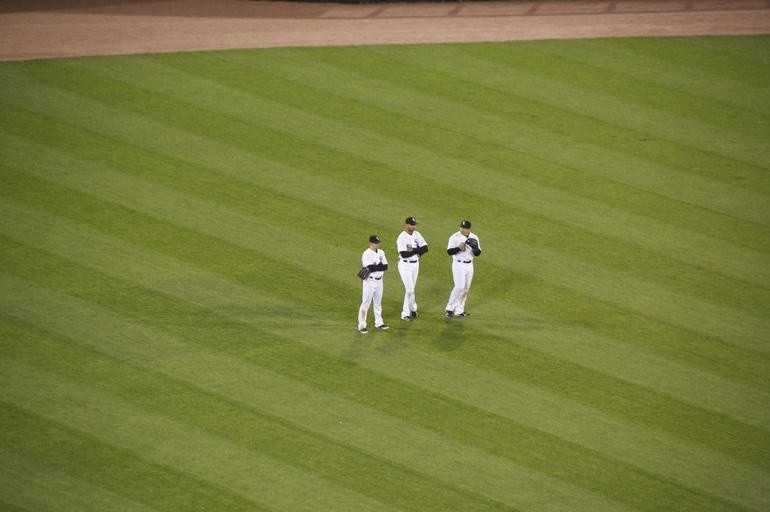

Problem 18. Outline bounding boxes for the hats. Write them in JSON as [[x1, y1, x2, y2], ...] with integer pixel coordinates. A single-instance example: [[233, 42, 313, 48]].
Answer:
[[369, 235, 382, 244], [459, 220, 471, 229], [405, 217, 418, 225]]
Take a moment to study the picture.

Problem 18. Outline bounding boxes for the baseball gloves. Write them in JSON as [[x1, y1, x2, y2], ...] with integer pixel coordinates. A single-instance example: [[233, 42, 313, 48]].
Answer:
[[465, 238, 478, 250], [358, 267, 369, 280]]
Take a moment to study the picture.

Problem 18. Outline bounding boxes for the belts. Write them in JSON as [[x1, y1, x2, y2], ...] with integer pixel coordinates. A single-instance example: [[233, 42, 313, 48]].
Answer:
[[370, 277, 381, 280], [404, 260, 417, 263], [457, 260, 471, 263]]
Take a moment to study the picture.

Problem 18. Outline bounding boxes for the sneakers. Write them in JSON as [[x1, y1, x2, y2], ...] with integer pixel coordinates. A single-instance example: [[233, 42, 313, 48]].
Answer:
[[359, 328, 368, 334], [379, 325, 390, 330], [401, 316, 413, 321], [456, 312, 471, 317], [445, 310, 452, 319], [412, 312, 418, 318]]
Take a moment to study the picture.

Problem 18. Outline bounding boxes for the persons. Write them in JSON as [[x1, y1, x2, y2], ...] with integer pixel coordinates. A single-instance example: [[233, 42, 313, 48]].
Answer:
[[357, 233, 390, 334], [443, 219, 482, 320], [396, 216, 429, 322]]
